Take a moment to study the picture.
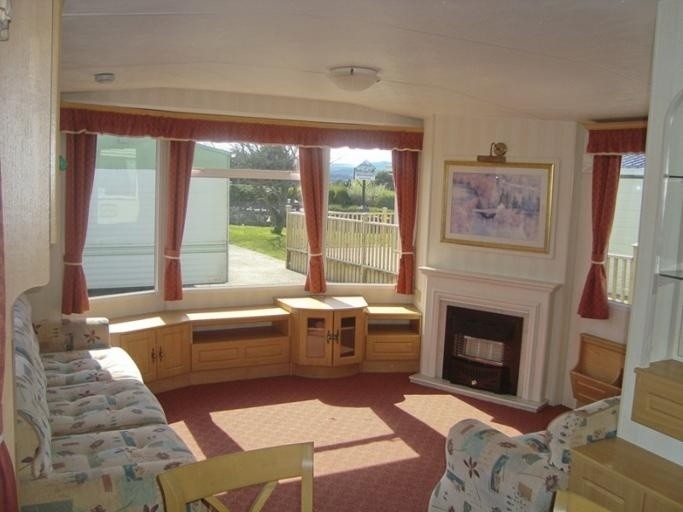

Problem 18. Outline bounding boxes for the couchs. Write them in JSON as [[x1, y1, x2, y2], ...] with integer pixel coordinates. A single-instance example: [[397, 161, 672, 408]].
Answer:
[[10, 294, 201, 511]]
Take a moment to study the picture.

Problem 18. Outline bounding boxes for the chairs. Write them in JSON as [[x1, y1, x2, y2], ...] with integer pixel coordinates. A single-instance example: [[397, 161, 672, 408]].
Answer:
[[159, 438, 317, 512], [426, 391, 619, 512]]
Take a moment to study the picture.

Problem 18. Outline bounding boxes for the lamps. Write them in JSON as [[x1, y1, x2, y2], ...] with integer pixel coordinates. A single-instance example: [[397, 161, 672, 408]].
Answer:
[[476, 140, 510, 165], [328, 65, 381, 96]]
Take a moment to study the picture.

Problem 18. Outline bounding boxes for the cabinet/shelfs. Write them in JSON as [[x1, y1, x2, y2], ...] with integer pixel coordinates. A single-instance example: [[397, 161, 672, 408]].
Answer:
[[616, 169, 683, 469], [106, 323, 191, 394], [274, 295, 367, 379], [189, 313, 292, 387], [568, 438, 683, 511], [364, 313, 421, 376]]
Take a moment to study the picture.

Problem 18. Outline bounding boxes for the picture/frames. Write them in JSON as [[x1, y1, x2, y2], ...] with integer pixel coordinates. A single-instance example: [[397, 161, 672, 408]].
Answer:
[[438, 158, 557, 254]]
